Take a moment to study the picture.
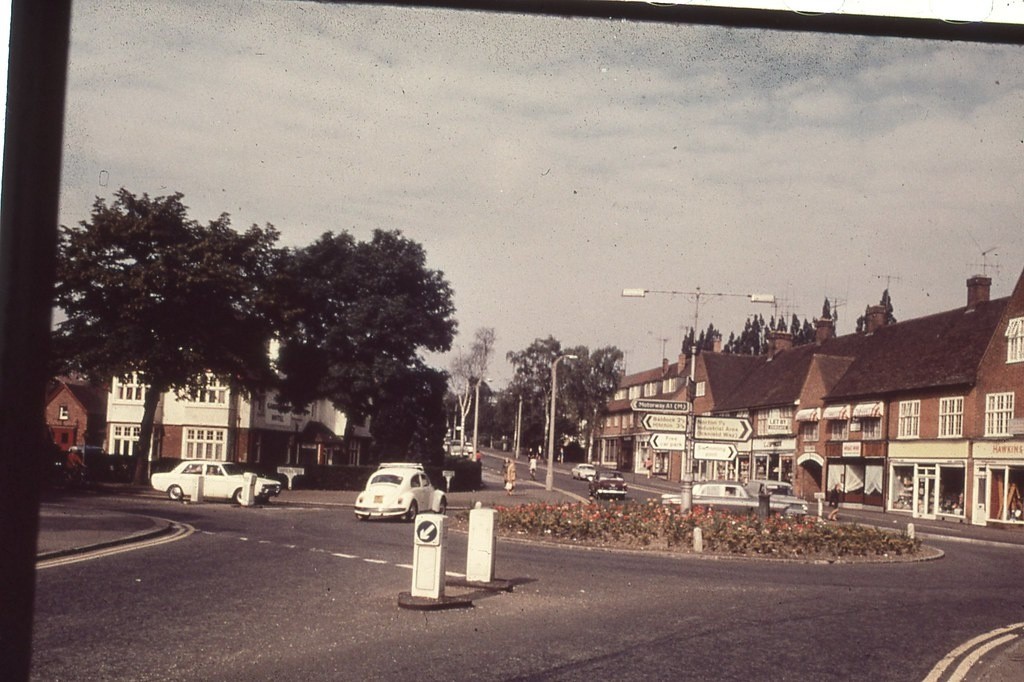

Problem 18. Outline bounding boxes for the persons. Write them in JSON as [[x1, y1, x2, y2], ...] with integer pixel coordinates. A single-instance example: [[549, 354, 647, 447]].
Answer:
[[528, 448, 533, 463], [500, 458, 510, 487], [529, 455, 536, 478], [67, 446, 86, 467], [508, 460, 516, 496], [646, 458, 652, 479], [827, 484, 841, 520], [208, 467, 215, 475], [477, 451, 481, 462]]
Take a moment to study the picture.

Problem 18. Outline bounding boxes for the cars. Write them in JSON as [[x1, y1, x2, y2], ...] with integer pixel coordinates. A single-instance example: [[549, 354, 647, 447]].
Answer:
[[354, 466, 448, 522], [571, 464, 598, 482], [152, 460, 281, 508], [442, 439, 474, 458], [588, 470, 629, 499], [660, 479, 788, 516]]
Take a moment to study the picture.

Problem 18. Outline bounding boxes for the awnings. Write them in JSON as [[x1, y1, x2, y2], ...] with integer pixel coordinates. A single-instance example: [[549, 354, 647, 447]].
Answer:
[[853, 400, 883, 418], [823, 404, 850, 420], [795, 407, 820, 421]]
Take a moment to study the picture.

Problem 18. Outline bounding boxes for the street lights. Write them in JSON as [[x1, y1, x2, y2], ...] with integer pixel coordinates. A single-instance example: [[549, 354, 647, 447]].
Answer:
[[545, 354, 578, 492], [473, 377, 493, 467], [623, 289, 775, 481]]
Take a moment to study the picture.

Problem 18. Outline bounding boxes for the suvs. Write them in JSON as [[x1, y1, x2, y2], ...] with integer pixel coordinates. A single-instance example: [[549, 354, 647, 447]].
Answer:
[[746, 480, 809, 516]]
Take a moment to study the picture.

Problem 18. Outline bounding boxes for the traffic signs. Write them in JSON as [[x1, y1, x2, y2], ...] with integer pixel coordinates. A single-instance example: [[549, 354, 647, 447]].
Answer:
[[632, 397, 752, 461]]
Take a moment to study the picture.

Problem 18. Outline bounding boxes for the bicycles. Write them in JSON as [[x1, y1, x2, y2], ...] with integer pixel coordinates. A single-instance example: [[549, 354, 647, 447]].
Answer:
[[58, 467, 86, 490]]
